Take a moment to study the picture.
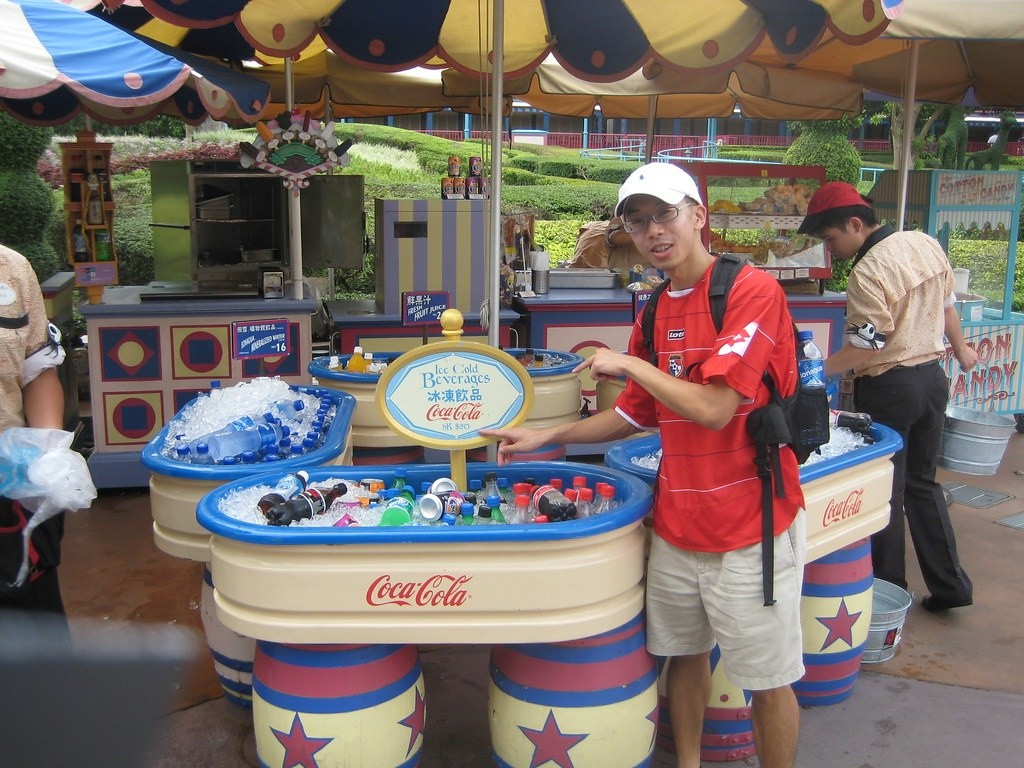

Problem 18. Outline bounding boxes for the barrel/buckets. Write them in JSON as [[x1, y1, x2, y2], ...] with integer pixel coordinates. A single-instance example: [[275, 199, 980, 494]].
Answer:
[[201, 561, 257, 708], [862, 577, 910, 665], [954, 293, 985, 321], [487, 608, 658, 768], [790, 535, 873, 705], [937, 361, 1016, 474], [655, 641, 757, 762], [251, 642, 426, 768]]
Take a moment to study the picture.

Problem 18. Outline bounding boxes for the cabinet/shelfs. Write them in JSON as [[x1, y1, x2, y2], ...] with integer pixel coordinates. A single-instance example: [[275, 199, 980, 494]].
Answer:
[[674, 162, 834, 296], [186, 168, 371, 274], [58, 131, 119, 266]]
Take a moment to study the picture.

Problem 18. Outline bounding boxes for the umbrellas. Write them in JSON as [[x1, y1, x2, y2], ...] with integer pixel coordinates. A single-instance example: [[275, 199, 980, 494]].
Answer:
[[0, 0, 236, 311], [93, 0, 1023, 383]]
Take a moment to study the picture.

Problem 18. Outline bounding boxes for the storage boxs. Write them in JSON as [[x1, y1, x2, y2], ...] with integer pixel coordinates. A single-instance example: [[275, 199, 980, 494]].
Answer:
[[549, 267, 622, 289]]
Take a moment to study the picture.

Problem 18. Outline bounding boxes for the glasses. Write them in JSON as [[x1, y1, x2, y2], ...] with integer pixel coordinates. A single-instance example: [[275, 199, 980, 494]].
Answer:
[[621, 202, 693, 234]]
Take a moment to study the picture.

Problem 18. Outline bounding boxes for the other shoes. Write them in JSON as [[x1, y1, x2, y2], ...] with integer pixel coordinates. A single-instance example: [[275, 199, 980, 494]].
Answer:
[[921, 593, 974, 613]]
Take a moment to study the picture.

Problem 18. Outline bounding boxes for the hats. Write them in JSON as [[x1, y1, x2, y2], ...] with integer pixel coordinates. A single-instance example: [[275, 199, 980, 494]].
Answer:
[[795, 181, 874, 233], [614, 160, 703, 220]]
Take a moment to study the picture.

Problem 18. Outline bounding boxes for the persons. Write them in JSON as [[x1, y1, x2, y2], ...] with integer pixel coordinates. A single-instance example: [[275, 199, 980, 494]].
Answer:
[[604, 214, 656, 289], [987, 131, 998, 149], [795, 181, 984, 611], [476, 161, 810, 767], [0, 245, 74, 615]]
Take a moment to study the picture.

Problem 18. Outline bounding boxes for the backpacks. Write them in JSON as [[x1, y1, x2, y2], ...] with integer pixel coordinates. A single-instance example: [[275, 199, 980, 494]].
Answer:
[[641, 250, 832, 466]]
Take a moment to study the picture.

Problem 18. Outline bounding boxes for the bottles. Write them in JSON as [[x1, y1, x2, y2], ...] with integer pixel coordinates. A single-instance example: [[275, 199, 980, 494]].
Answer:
[[68, 156, 111, 262], [797, 327, 826, 388], [258, 469, 615, 526], [954, 267, 968, 293], [173, 378, 336, 464], [496, 345, 543, 367], [828, 410, 873, 451], [327, 346, 393, 375]]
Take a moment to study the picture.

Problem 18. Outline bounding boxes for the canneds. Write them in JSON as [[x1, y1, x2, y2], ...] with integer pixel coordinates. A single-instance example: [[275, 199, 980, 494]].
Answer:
[[418, 477, 465, 521], [441, 156, 489, 200], [332, 478, 386, 527]]
